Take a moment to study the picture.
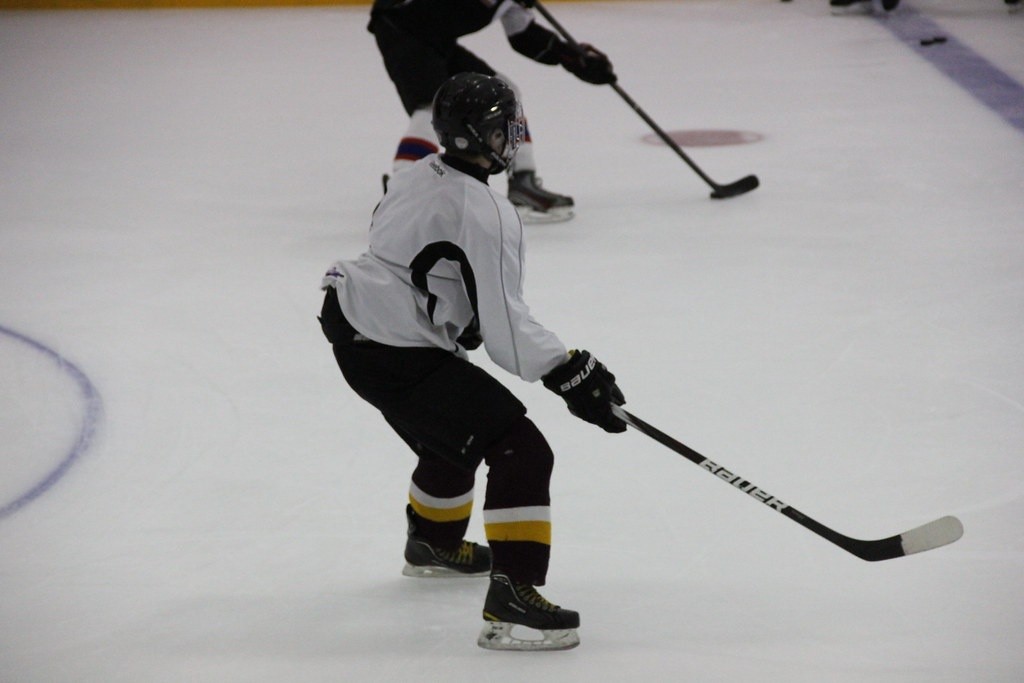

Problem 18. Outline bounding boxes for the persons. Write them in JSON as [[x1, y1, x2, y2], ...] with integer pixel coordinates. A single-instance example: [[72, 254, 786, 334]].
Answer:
[[315, 70, 629, 632], [365, 0, 618, 229]]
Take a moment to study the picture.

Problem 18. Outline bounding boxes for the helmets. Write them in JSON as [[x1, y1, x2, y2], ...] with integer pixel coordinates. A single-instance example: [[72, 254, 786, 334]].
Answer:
[[430, 72, 516, 158]]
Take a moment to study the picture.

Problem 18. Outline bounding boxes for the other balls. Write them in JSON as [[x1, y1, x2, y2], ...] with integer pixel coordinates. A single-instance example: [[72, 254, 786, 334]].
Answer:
[[933, 37, 946, 42], [921, 39, 933, 45], [710, 191, 725, 197]]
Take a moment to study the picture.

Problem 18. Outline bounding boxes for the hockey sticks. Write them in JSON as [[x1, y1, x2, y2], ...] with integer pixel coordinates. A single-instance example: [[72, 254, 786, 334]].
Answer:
[[611, 385, 964, 563], [529, 0, 760, 199]]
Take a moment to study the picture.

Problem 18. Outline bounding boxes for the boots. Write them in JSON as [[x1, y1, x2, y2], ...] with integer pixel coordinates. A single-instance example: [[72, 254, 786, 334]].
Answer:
[[402, 504, 491, 577], [507, 170, 575, 223], [478, 573, 580, 650]]
